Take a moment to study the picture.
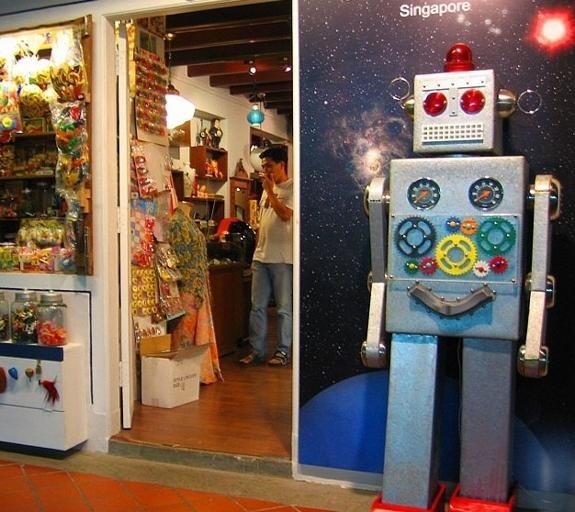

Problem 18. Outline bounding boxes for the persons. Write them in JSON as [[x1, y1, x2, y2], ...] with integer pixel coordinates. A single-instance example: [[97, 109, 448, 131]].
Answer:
[[237, 145, 293, 368], [166, 200, 228, 385]]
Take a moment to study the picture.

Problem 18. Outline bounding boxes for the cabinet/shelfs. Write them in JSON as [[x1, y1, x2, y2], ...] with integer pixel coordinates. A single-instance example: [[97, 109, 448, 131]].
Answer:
[[169, 145, 229, 243]]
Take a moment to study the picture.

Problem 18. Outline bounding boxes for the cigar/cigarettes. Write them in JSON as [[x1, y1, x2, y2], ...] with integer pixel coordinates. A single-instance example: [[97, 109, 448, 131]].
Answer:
[[258, 174, 266, 177]]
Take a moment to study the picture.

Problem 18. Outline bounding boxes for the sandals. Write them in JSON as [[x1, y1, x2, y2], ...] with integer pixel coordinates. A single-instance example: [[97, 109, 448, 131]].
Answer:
[[268, 349, 291, 366], [234, 350, 269, 367]]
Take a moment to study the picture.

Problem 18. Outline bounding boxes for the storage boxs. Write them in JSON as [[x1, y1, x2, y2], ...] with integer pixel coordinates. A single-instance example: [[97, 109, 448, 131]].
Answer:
[[138, 335, 212, 410]]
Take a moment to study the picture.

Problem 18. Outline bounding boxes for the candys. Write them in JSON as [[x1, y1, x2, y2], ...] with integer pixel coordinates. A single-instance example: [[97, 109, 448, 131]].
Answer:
[[0, 305, 67, 346]]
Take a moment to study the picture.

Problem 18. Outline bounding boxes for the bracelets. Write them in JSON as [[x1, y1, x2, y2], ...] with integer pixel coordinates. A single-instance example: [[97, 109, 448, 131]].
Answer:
[[264, 193, 278, 210]]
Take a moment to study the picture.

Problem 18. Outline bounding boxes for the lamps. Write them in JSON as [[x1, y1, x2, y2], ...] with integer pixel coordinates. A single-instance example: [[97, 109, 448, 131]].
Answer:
[[161, 33, 197, 130]]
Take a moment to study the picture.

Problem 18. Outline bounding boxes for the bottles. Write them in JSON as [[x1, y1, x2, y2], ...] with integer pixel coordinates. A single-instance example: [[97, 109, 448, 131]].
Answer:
[[35, 288, 69, 347], [0, 290, 11, 342], [9, 287, 39, 345]]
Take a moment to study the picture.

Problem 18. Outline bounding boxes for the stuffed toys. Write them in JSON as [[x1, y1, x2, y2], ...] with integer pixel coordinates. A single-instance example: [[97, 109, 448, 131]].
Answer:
[[209, 117, 224, 148]]
